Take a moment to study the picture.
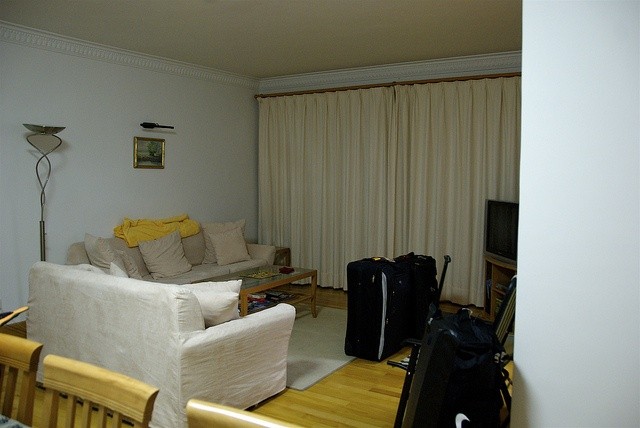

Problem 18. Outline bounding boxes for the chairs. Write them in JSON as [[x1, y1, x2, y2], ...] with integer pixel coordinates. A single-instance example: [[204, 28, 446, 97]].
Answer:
[[43, 352, 157, 427], [185, 398, 302, 428], [1, 329, 44, 425]]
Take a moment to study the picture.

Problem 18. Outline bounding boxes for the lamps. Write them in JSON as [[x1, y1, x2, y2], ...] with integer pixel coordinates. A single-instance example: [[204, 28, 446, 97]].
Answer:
[[23, 122, 66, 260]]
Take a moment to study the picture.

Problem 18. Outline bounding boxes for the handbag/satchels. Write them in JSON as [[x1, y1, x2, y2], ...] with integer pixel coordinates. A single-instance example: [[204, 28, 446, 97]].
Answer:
[[412, 308, 513, 427]]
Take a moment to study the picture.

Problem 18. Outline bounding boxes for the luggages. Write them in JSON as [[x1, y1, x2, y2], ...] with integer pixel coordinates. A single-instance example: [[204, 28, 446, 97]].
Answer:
[[393, 251, 441, 341], [345, 256, 410, 362]]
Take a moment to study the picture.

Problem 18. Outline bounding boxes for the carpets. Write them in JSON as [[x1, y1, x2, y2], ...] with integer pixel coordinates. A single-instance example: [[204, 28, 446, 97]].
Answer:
[[280, 300, 365, 392]]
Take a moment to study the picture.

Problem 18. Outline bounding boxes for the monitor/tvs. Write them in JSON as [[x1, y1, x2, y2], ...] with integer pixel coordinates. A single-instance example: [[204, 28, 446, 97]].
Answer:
[[484, 198, 519, 265]]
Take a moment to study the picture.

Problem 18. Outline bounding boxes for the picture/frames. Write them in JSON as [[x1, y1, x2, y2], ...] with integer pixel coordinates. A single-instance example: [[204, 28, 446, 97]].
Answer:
[[133, 136, 166, 171]]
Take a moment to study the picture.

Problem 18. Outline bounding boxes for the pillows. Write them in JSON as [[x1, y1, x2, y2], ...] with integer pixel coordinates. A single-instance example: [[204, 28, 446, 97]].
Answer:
[[211, 227, 251, 264], [193, 290, 241, 322], [200, 218, 250, 267], [137, 228, 193, 280], [107, 259, 129, 280], [189, 278, 244, 299], [83, 234, 128, 278], [116, 251, 144, 280]]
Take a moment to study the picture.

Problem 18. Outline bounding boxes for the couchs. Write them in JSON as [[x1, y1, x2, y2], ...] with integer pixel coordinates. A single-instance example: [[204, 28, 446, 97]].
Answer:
[[27, 261, 298, 427], [66, 215, 291, 285]]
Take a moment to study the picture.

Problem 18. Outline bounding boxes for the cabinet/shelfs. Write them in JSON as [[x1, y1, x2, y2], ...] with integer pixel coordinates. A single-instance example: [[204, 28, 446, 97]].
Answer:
[[477, 256, 516, 323]]
